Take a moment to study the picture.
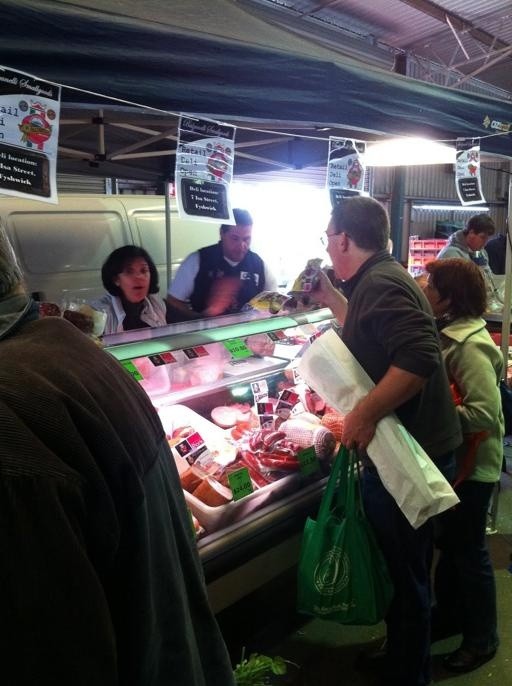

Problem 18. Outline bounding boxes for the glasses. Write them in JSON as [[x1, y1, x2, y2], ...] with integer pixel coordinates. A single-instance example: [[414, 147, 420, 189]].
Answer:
[[319, 230, 352, 244]]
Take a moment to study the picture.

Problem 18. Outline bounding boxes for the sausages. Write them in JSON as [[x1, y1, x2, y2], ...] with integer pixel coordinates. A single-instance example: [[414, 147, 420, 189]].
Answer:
[[239, 428, 299, 488]]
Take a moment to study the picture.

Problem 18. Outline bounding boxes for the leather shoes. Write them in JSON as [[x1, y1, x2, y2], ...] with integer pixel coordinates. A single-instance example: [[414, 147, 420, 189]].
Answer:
[[445, 647, 498, 673]]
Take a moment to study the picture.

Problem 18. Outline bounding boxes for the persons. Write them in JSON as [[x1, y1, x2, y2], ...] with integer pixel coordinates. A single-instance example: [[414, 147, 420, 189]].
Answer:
[[88, 245, 168, 338], [292, 195, 464, 684], [415, 258, 506, 676], [1, 217, 237, 681], [434, 211, 495, 274], [165, 208, 280, 318]]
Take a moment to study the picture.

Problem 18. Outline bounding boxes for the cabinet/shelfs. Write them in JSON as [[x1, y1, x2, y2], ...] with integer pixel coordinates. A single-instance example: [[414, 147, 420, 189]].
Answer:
[[97, 305, 364, 613]]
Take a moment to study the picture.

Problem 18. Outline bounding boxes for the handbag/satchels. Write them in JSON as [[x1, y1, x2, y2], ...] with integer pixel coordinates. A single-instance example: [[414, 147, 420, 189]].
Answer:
[[295, 444, 398, 629]]
